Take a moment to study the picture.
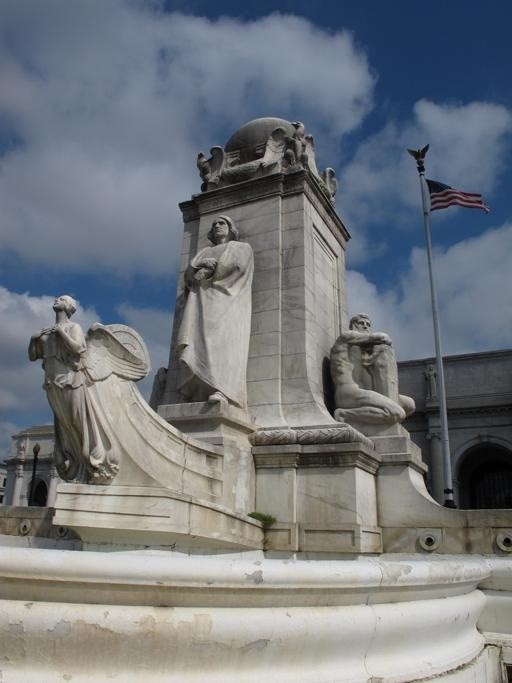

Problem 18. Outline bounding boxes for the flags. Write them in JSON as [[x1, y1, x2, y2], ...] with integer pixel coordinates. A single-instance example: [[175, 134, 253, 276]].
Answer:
[[425, 178, 490, 215]]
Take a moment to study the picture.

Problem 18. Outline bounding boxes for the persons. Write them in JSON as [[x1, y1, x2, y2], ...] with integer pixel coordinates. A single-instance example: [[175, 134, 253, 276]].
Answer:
[[27, 293, 122, 485], [173, 214, 255, 410], [329, 314, 416, 426]]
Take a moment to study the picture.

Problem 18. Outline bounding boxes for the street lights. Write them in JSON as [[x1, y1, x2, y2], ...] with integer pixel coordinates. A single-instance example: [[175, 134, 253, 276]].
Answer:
[[29, 443, 40, 505]]
